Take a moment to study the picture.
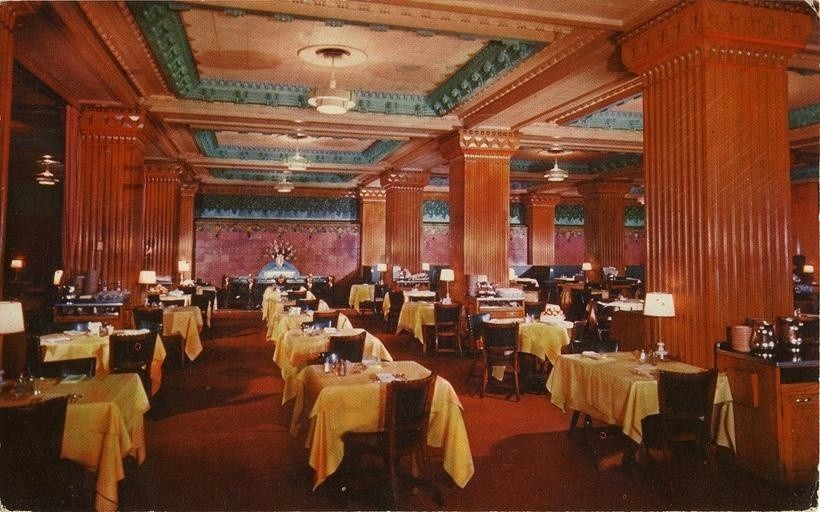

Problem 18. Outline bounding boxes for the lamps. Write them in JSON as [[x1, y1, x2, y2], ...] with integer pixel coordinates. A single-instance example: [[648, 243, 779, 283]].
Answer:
[[33, 166, 59, 185], [307, 49, 358, 114], [544, 148, 568, 181], [644, 292, 677, 362], [272, 173, 295, 193], [285, 134, 313, 172], [34, 148, 60, 166]]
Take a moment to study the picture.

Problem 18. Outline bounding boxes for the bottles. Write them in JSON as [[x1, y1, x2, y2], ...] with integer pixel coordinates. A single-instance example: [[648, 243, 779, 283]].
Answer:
[[324, 359, 346, 377], [15, 375, 43, 399], [524, 313, 530, 324], [116, 281, 121, 292], [98, 280, 109, 292], [640, 349, 646, 360], [750, 316, 805, 362]]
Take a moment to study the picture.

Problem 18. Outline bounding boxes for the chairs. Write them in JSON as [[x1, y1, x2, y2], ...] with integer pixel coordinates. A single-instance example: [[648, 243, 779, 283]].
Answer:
[[313, 309, 340, 329], [330, 331, 365, 363], [106, 324, 162, 435], [222, 275, 336, 310], [342, 369, 445, 511], [2, 393, 86, 511], [478, 320, 521, 400], [2, 264, 220, 377], [346, 264, 644, 358]]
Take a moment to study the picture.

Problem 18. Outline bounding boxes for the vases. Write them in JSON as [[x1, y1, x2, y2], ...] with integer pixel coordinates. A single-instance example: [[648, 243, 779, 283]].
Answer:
[[275, 255, 285, 268]]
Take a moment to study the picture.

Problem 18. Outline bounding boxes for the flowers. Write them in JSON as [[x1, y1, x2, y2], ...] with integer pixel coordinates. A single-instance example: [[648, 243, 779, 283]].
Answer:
[[263, 239, 297, 261]]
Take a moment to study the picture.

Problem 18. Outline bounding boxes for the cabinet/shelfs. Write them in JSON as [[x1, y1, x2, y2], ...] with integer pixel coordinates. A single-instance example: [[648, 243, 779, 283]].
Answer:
[[715, 341, 820, 492]]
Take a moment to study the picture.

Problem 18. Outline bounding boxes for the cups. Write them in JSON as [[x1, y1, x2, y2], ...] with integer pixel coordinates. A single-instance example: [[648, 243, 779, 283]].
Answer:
[[106, 325, 114, 335]]
